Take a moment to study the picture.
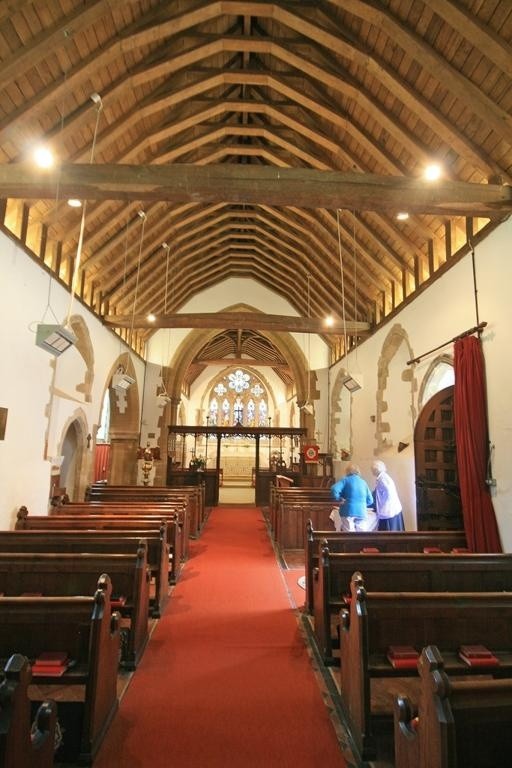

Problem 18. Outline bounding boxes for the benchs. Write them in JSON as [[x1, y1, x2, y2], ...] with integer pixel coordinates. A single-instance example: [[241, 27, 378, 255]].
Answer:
[[266, 477, 343, 553]]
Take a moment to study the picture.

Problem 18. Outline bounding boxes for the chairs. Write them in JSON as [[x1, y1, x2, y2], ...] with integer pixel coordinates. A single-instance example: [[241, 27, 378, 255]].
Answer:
[[420, 486, 462, 530]]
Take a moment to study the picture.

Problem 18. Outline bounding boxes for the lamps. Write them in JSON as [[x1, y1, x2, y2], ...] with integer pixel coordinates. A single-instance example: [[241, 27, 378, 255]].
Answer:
[[335, 202, 368, 397], [299, 272, 315, 417], [110, 199, 148, 394], [33, 27, 104, 357]]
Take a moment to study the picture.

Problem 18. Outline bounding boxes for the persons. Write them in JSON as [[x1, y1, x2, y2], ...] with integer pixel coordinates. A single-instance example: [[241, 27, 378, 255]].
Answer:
[[372, 460, 405, 531], [332, 464, 373, 532]]
